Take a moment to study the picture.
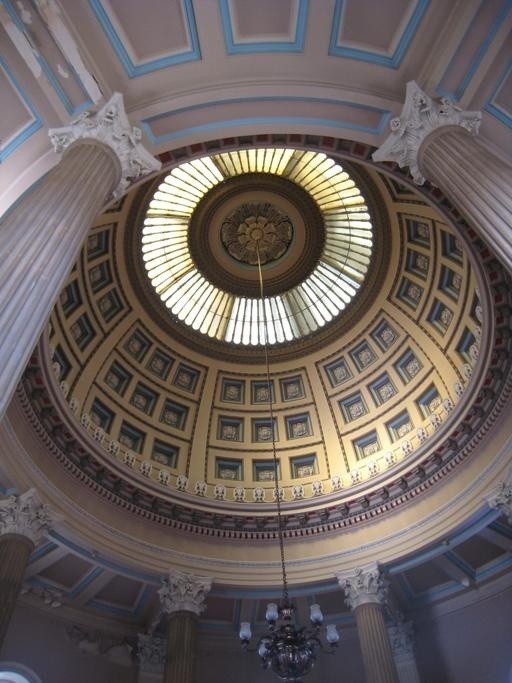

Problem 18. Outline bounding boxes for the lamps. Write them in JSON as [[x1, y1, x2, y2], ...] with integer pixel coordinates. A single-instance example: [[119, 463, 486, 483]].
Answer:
[[239, 240, 338, 681]]
